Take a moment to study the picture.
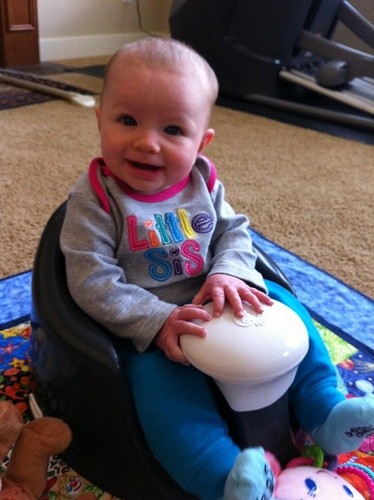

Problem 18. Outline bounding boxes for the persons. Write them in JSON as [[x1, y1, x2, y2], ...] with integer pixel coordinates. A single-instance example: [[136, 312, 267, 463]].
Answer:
[[60, 37, 374, 499]]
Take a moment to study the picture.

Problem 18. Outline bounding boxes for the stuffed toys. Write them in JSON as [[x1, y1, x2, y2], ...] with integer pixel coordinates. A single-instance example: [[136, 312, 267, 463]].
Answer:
[[265, 451, 367, 499], [0, 400, 72, 500]]
